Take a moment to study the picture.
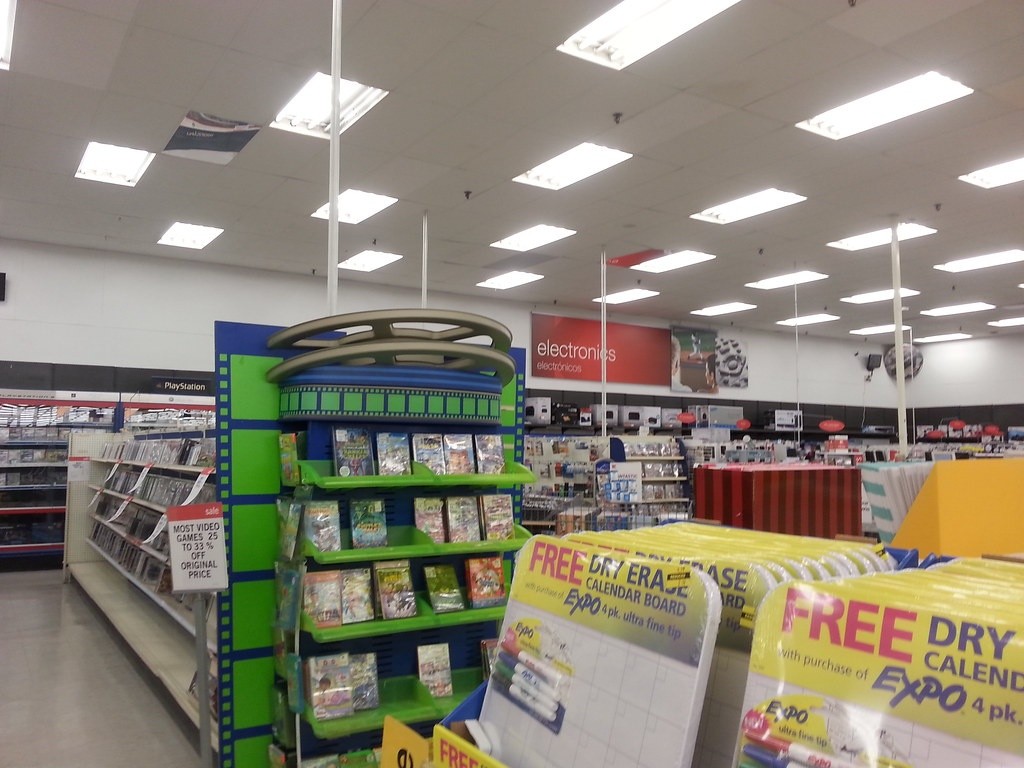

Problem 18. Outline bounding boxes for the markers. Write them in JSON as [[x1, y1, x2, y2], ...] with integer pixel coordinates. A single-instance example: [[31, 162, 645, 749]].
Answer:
[[492, 643, 564, 723], [738, 729, 862, 768]]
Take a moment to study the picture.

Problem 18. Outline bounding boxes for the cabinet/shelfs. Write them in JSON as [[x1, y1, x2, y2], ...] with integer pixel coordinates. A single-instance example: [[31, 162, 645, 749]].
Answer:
[[3, 305, 1023, 768]]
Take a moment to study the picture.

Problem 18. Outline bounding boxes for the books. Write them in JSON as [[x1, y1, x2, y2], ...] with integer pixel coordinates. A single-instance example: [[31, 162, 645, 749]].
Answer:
[[524, 439, 685, 509], [0, 425, 216, 623], [304, 430, 514, 722]]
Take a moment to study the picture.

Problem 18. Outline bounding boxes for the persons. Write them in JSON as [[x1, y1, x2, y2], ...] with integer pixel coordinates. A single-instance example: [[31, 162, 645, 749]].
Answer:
[[688, 332, 705, 362], [672, 336, 692, 392], [697, 354, 718, 393]]
